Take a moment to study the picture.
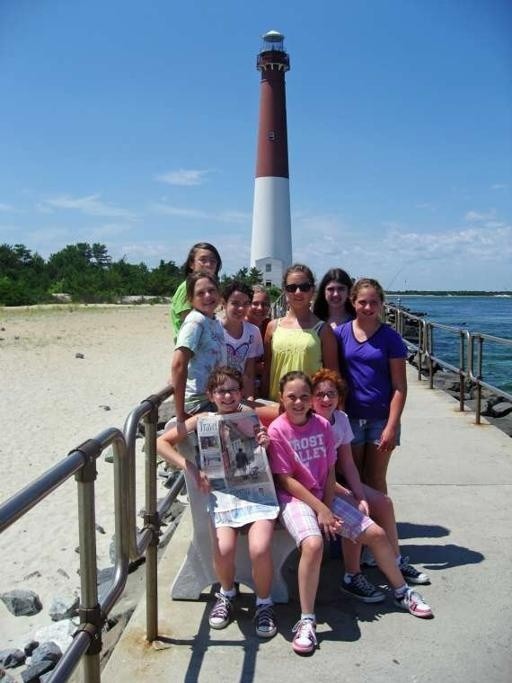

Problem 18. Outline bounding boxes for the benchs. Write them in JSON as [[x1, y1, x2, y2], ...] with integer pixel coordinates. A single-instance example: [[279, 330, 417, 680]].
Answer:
[[164, 414, 298, 603]]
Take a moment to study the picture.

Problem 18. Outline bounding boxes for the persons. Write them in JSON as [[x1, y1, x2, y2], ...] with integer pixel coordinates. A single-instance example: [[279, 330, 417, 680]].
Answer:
[[171, 271, 279, 428], [171, 243, 220, 343], [261, 265, 336, 403], [156, 368, 277, 638], [314, 268, 357, 327], [243, 284, 272, 343], [336, 278, 410, 564], [266, 371, 432, 653], [217, 281, 265, 403], [310, 369, 431, 603]]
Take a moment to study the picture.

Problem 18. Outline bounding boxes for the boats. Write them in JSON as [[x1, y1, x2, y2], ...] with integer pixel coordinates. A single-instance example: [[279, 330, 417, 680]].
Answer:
[[383, 302, 431, 341]]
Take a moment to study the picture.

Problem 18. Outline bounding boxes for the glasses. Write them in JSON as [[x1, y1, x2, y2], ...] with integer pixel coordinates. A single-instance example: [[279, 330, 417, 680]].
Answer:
[[315, 390, 337, 398], [214, 387, 240, 394], [285, 282, 311, 292]]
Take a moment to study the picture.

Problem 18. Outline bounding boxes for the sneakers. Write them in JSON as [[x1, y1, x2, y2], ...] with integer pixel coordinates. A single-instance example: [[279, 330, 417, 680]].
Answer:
[[337, 548, 433, 618], [292, 617, 318, 654], [209, 591, 239, 629], [255, 604, 279, 638]]
